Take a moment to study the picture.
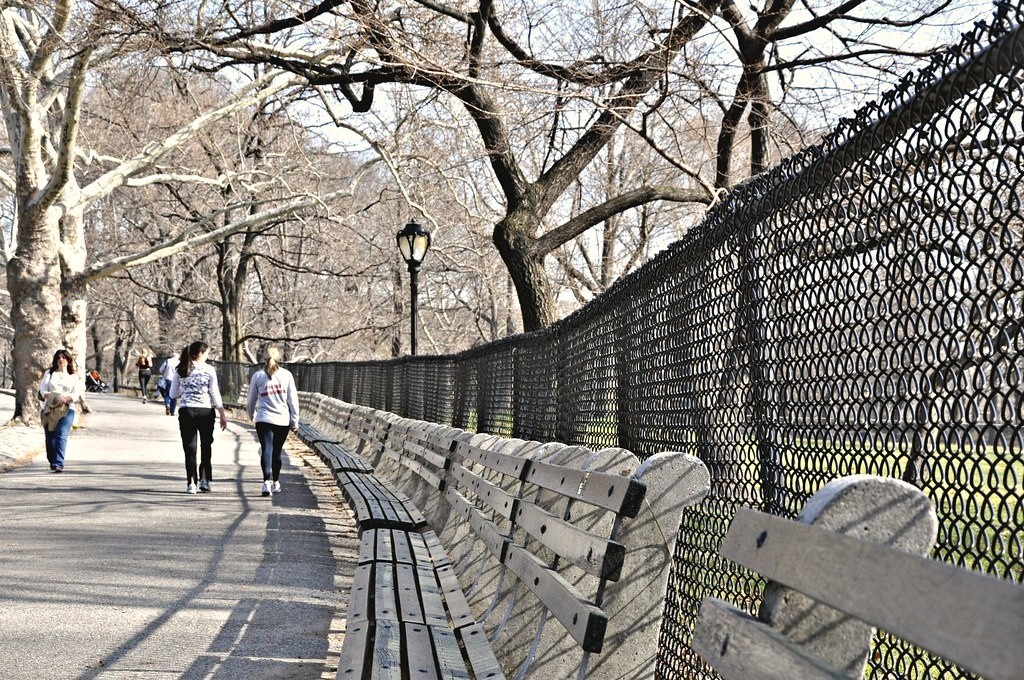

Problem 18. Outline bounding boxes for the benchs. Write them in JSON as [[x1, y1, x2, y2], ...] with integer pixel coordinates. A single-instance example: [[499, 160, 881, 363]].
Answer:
[[239, 383, 1024, 680]]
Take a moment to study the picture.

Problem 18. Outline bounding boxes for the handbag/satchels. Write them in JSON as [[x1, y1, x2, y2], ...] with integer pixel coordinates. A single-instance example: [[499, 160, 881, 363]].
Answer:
[[155, 375, 167, 389], [38, 369, 52, 402]]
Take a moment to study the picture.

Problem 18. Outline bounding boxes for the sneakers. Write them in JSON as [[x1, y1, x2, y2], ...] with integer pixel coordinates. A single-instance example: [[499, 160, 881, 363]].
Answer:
[[272, 480, 281, 492], [186, 484, 197, 493], [199, 480, 211, 491], [262, 480, 273, 495]]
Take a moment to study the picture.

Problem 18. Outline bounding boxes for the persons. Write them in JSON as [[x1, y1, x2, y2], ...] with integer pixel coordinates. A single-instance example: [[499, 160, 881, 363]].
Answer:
[[246, 348, 299, 495], [136, 348, 153, 404], [169, 341, 227, 494], [37, 349, 80, 473], [159, 352, 180, 416], [85, 368, 109, 392]]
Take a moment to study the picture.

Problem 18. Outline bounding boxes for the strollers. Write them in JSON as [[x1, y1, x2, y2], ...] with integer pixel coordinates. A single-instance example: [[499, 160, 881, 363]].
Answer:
[[86, 370, 109, 393]]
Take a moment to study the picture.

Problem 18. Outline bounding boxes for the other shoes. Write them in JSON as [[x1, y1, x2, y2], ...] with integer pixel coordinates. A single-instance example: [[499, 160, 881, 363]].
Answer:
[[171, 413, 174, 416], [143, 395, 147, 403], [166, 404, 170, 415], [55, 466, 63, 472]]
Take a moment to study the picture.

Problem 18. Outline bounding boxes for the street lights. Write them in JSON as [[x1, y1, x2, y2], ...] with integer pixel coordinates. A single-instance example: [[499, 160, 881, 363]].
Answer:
[[396, 218, 431, 356]]
